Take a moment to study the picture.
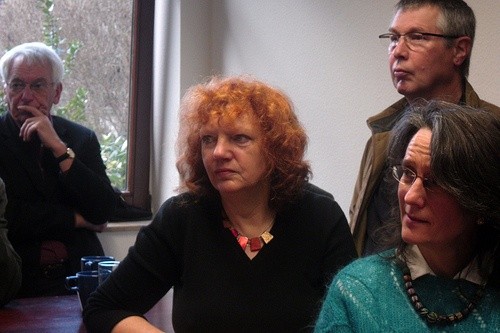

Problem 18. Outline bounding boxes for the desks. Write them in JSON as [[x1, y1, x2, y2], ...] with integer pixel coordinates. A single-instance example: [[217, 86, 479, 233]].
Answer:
[[0, 289, 173, 333]]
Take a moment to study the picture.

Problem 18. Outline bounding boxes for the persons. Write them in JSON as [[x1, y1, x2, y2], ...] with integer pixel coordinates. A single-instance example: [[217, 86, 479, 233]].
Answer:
[[82, 75, 359, 333], [313, 98, 500, 333], [349, 0, 500, 258], [0, 42, 122, 298]]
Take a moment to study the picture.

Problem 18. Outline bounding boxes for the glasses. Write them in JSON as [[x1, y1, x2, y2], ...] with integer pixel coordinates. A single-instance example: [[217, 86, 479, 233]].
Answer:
[[10, 80, 48, 92], [391, 165, 440, 191], [379, 31, 459, 42]]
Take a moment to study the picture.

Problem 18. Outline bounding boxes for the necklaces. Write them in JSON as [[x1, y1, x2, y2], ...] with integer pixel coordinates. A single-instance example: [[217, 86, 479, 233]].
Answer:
[[223, 218, 274, 252], [398, 258, 481, 327]]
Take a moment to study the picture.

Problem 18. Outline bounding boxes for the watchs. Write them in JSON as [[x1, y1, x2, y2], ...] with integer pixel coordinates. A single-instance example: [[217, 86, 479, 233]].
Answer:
[[56, 148, 76, 164]]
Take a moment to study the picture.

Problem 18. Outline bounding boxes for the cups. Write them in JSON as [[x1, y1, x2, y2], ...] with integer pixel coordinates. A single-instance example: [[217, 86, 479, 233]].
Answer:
[[98, 261, 120, 286], [80, 256, 114, 271], [66, 271, 98, 311]]
